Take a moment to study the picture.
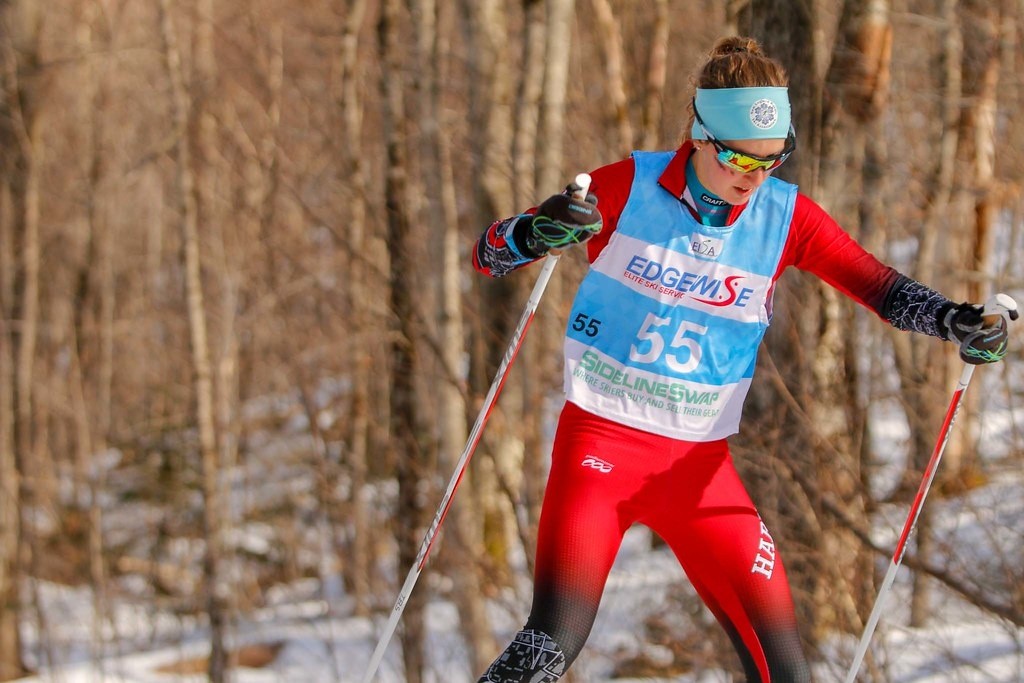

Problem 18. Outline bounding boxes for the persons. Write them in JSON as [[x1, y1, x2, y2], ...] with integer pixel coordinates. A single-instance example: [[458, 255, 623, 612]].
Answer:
[[471, 37, 1009, 683]]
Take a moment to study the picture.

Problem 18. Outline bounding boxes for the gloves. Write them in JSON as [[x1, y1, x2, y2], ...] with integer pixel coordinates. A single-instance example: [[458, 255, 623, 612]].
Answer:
[[935, 301, 1008, 366], [512, 195, 603, 260]]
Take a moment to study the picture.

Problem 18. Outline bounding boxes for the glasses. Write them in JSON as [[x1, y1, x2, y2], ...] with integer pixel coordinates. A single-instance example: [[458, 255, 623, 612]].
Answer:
[[715, 133, 797, 172]]
